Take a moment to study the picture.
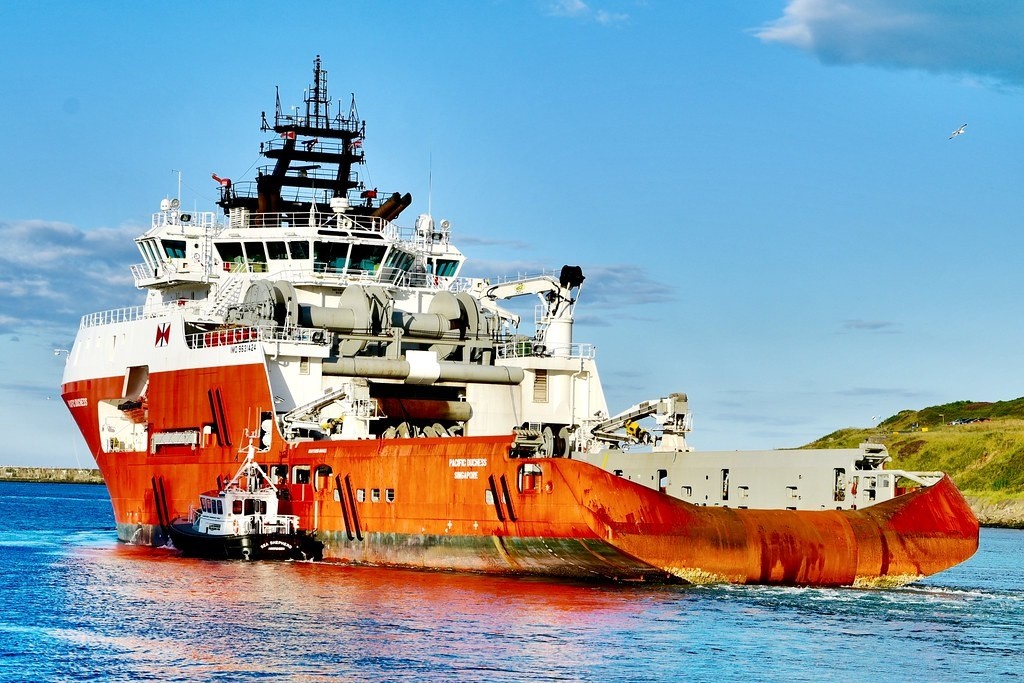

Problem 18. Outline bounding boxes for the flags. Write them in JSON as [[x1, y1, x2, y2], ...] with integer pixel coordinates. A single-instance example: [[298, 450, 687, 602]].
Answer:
[[348, 139, 363, 149], [872, 416, 876, 420], [280, 131, 294, 139]]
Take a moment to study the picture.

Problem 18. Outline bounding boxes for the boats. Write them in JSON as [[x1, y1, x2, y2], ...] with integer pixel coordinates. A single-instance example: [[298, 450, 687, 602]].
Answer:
[[52, 54, 980, 587], [165, 407, 325, 562]]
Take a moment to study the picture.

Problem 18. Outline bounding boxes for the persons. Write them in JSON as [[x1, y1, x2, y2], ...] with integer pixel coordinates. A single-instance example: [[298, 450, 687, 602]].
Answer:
[[837, 478, 845, 499]]
